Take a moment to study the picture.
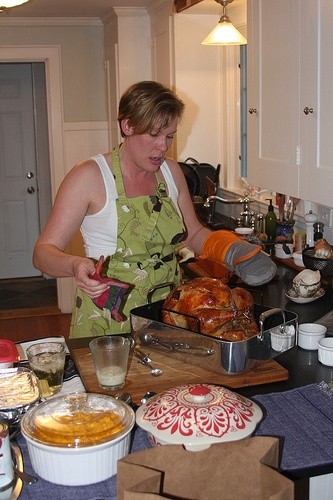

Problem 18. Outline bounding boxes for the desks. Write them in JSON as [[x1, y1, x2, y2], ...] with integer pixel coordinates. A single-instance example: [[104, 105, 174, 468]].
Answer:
[[172, 203, 332, 338], [0, 332, 291, 431]]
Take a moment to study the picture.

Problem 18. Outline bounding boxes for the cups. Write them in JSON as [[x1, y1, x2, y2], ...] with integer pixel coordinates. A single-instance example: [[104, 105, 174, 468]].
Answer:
[[89, 336, 132, 391], [275, 218, 295, 245]]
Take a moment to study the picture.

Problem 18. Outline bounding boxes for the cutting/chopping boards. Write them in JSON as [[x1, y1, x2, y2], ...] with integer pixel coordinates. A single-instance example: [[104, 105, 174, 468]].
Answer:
[[70, 342, 289, 409]]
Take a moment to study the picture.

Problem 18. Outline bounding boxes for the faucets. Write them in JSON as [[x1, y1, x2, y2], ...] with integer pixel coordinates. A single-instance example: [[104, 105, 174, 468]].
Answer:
[[203, 193, 254, 226]]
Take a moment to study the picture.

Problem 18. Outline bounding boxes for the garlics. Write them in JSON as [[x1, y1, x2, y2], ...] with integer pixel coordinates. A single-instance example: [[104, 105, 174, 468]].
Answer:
[[313, 248, 331, 269]]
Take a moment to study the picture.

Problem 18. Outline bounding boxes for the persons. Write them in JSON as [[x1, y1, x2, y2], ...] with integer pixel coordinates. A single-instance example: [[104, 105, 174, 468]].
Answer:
[[33, 80, 276, 341]]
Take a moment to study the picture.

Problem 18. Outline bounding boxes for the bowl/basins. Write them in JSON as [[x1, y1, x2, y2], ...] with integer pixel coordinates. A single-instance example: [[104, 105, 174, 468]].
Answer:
[[297, 323, 328, 350], [20, 392, 135, 486], [293, 251, 305, 268], [270, 325, 294, 352], [135, 382, 264, 452], [316, 338, 333, 367], [274, 243, 294, 259]]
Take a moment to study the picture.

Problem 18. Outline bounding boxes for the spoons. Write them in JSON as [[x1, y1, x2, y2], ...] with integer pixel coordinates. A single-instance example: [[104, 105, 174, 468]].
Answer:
[[133, 349, 162, 377], [176, 347, 217, 355]]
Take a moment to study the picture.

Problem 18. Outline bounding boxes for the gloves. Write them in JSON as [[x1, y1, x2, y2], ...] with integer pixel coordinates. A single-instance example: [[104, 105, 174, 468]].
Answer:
[[200, 230, 278, 288]]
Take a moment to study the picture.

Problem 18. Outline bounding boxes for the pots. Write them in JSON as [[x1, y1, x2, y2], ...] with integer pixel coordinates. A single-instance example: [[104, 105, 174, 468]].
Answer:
[[177, 157, 202, 202]]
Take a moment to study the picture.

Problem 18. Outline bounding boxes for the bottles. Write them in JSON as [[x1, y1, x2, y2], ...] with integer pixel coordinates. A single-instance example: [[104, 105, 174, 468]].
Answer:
[[26, 341, 64, 398]]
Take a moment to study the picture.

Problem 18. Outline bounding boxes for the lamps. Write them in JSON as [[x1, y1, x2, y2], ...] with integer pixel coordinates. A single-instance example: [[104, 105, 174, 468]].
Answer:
[[199, 0, 248, 48]]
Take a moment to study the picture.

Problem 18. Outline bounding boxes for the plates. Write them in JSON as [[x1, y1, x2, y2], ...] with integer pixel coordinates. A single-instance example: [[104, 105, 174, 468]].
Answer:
[[284, 286, 325, 303]]
